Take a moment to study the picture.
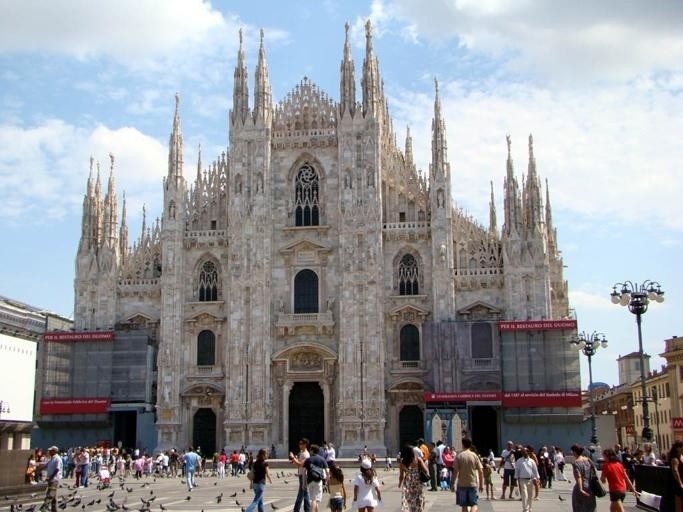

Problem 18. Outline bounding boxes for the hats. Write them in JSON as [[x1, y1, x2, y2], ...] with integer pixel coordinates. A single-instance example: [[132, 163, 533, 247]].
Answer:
[[360, 459, 371, 470], [47, 446, 58, 453]]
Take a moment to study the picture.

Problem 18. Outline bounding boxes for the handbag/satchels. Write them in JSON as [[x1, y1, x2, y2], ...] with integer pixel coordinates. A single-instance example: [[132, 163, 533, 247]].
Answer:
[[418, 462, 431, 483], [309, 462, 323, 482], [634, 491, 662, 512], [587, 459, 605, 498], [246, 466, 255, 481]]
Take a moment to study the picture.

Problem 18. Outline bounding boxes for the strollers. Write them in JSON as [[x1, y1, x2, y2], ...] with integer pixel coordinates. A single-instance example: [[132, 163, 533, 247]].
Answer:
[[96, 464, 112, 492]]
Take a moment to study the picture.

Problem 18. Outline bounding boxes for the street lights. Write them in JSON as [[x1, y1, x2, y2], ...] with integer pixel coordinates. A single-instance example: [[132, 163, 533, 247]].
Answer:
[[0, 399, 11, 417], [607, 278, 666, 462], [600, 405, 638, 416], [567, 329, 608, 450]]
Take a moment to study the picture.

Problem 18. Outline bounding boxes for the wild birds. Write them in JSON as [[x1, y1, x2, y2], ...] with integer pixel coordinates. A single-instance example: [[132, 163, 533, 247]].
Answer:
[[4, 474, 192, 512], [236, 499, 240, 506], [242, 488, 245, 493], [270, 502, 279, 512], [381, 481, 384, 486], [559, 495, 566, 502], [229, 492, 237, 498], [241, 507, 245, 512], [216, 491, 223, 504], [284, 480, 288, 484], [214, 482, 217, 486], [201, 509, 204, 512], [276, 470, 293, 479]]
